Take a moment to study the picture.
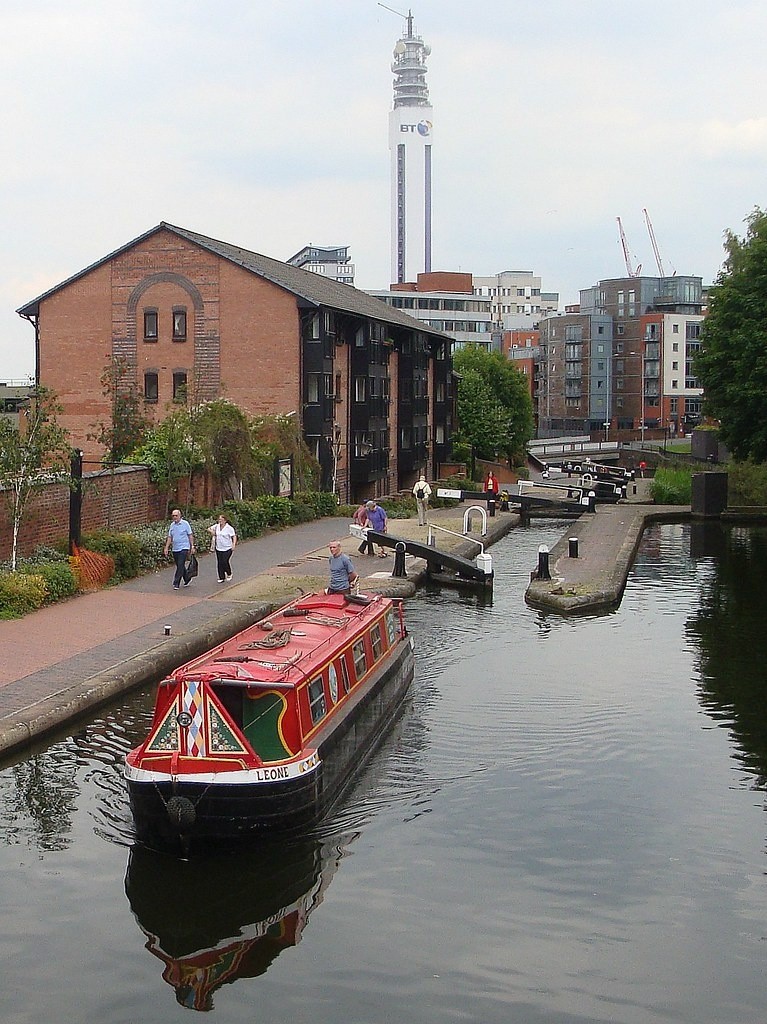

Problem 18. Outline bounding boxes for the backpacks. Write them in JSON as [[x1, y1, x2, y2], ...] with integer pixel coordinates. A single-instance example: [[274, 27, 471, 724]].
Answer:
[[417, 483, 427, 498]]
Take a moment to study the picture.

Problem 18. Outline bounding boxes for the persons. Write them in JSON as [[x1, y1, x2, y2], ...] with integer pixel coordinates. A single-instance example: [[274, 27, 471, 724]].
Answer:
[[208, 515, 237, 582], [328, 540, 356, 595], [164, 510, 196, 590], [413, 475, 431, 526], [353, 499, 388, 558], [484, 471, 499, 510]]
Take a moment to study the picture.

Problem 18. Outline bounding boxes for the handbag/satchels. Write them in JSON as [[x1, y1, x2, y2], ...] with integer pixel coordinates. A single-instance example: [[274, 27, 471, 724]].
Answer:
[[188, 554, 198, 577], [209, 536, 216, 552], [353, 511, 358, 518]]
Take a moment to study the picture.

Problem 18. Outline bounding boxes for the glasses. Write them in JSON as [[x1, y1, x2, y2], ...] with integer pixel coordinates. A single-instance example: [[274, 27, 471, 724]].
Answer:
[[172, 515, 178, 517]]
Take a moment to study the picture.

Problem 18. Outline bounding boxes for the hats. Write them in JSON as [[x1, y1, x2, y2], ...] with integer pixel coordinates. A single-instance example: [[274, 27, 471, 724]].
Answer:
[[367, 501, 376, 508], [419, 476, 426, 480]]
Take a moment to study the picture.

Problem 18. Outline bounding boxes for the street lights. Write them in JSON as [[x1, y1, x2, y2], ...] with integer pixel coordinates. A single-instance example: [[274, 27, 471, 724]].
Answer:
[[606, 354, 618, 441], [630, 352, 644, 450]]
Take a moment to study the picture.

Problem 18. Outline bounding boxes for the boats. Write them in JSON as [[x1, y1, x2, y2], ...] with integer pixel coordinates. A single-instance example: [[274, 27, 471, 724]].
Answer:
[[123, 581, 415, 862], [124, 832, 361, 1014]]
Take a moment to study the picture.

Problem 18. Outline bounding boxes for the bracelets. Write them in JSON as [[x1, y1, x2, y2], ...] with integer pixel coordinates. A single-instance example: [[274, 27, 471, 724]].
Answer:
[[191, 546, 194, 548]]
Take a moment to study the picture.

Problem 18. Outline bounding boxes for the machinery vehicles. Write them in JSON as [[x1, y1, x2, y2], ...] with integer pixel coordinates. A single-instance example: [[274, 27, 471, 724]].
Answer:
[[616, 217, 642, 278], [643, 209, 676, 278]]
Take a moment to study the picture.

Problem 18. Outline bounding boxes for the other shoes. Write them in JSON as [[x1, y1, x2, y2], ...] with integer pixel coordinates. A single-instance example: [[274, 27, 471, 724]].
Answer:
[[217, 579, 225, 583], [173, 584, 179, 590], [377, 551, 388, 557], [368, 552, 376, 556], [358, 547, 365, 554], [184, 578, 192, 586], [227, 573, 233, 581]]
